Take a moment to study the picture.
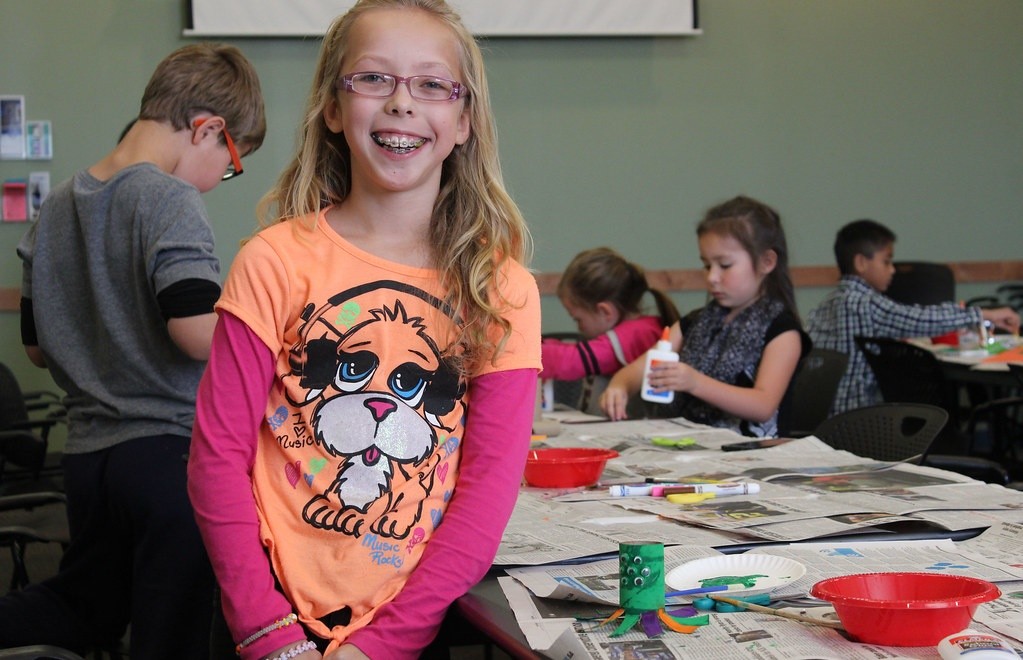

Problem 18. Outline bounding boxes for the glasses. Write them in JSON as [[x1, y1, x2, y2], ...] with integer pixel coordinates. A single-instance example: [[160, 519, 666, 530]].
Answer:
[[192, 117, 244, 181], [334, 70, 468, 102]]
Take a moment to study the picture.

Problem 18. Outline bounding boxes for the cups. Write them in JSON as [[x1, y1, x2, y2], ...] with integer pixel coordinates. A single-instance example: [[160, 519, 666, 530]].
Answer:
[[619, 541, 665, 613]]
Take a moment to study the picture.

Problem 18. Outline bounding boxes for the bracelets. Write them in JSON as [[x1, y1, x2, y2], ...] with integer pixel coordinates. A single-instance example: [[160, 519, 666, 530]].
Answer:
[[266, 641, 317, 660], [236, 612, 298, 653]]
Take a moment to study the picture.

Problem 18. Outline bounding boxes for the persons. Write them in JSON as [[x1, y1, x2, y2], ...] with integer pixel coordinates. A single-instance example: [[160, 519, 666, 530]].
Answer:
[[539, 248, 679, 380], [186, 0, 543, 660], [16, 42, 267, 660], [806, 219, 1021, 419], [599, 194, 813, 439]]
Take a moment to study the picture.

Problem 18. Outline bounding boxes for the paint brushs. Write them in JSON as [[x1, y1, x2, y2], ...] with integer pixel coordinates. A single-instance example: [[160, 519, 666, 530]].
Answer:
[[705, 594, 844, 629]]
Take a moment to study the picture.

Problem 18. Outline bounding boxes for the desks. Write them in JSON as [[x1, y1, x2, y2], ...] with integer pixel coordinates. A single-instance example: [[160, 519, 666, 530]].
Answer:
[[449, 400, 1023, 660], [895, 332, 1023, 489]]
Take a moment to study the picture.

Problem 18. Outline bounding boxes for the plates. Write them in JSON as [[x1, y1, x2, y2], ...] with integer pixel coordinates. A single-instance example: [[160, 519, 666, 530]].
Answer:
[[665, 553, 806, 598]]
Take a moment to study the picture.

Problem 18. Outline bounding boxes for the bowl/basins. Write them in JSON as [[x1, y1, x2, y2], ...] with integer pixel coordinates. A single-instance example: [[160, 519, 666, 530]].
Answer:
[[809, 569, 1002, 646], [931, 331, 959, 346], [523, 448, 620, 488]]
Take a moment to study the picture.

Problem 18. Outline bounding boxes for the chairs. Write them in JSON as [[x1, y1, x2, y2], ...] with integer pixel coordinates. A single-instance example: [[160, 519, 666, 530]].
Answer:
[[0, 361, 83, 660], [880, 262, 955, 305], [784, 336, 1023, 489]]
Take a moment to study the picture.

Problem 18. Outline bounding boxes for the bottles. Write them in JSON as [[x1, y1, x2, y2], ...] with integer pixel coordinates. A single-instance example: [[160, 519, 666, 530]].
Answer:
[[640, 327, 679, 404], [937, 628, 1021, 660]]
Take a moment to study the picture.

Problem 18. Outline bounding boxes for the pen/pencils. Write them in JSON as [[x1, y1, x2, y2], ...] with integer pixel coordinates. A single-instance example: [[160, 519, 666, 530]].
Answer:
[[646, 477, 723, 483]]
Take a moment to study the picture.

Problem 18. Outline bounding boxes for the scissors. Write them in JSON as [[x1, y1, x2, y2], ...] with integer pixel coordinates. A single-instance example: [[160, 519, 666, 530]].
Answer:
[[692, 591, 807, 614], [653, 437, 695, 451], [668, 492, 735, 504]]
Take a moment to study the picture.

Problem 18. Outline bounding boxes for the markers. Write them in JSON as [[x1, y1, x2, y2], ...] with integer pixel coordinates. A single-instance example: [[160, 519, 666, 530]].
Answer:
[[609, 484, 672, 496], [663, 482, 759, 497], [653, 486, 681, 497]]
